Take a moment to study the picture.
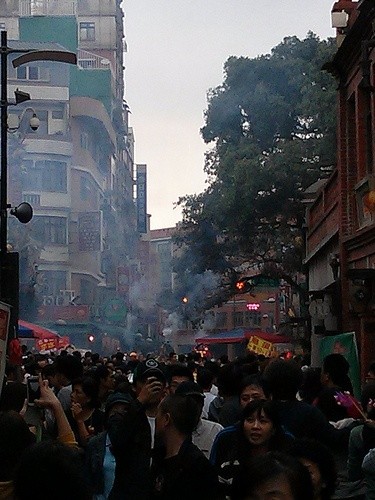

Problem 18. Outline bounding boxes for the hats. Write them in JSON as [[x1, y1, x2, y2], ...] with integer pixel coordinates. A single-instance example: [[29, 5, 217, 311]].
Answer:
[[175, 380, 205, 398], [133, 358, 166, 384], [105, 392, 132, 412]]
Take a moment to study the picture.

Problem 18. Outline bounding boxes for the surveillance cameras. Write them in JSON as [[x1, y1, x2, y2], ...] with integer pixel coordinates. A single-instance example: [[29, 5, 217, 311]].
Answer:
[[30, 117, 39, 131]]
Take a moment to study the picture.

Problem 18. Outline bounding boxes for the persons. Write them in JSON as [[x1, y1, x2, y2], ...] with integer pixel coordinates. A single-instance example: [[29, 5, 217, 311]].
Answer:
[[0, 335, 375, 500]]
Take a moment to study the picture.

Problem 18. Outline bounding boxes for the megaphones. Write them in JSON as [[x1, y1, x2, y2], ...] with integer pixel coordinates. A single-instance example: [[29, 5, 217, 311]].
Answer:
[[12, 202, 33, 225]]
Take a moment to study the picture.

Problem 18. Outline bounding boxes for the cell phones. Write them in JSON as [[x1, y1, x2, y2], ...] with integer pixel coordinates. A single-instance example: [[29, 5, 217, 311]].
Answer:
[[147, 377, 157, 384], [27, 376, 43, 406]]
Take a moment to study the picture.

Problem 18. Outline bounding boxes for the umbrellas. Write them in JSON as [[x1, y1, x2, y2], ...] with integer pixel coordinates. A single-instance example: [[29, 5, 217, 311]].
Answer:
[[194, 326, 291, 344], [16, 320, 62, 339]]
